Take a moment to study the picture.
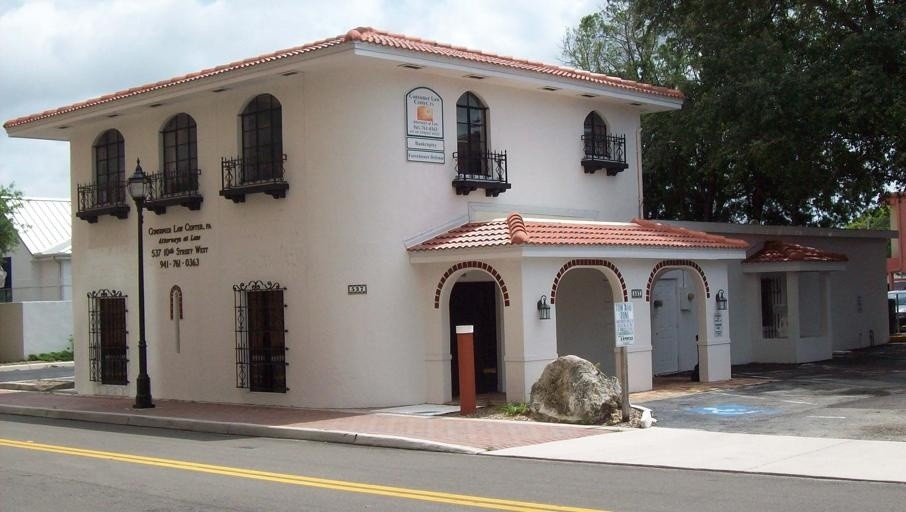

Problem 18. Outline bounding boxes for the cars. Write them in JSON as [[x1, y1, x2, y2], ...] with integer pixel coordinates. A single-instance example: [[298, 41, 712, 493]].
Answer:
[[887, 290, 906, 326]]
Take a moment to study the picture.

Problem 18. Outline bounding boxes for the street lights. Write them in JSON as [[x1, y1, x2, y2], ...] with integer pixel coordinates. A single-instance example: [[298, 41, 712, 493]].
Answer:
[[125, 159, 156, 409]]
